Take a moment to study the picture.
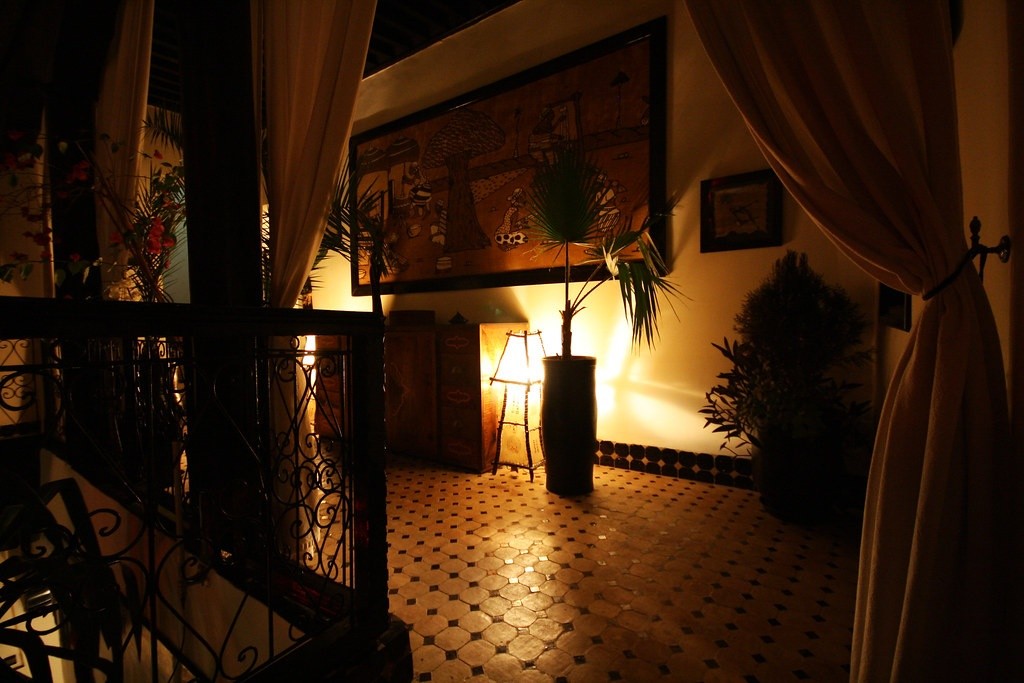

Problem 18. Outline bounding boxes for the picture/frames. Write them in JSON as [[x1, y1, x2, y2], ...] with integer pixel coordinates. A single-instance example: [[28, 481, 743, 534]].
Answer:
[[334, 13, 678, 297], [695, 168, 782, 254]]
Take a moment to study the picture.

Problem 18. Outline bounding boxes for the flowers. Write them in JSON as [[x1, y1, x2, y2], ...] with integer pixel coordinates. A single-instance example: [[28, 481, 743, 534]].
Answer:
[[1, 122, 185, 272]]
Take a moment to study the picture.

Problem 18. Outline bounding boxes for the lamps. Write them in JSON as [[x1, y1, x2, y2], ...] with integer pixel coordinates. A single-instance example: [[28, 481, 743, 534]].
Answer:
[[489, 327, 550, 483]]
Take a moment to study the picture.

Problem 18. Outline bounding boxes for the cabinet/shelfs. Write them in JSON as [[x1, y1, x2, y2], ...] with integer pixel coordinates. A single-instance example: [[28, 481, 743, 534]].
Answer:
[[312, 321, 534, 475]]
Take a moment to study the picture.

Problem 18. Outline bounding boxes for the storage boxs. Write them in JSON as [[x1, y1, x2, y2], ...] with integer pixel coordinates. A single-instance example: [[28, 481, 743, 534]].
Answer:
[[388, 310, 434, 326]]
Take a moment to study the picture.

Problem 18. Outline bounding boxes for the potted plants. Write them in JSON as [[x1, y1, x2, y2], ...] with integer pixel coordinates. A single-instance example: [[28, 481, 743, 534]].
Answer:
[[699, 251, 877, 526], [522, 127, 684, 497]]
